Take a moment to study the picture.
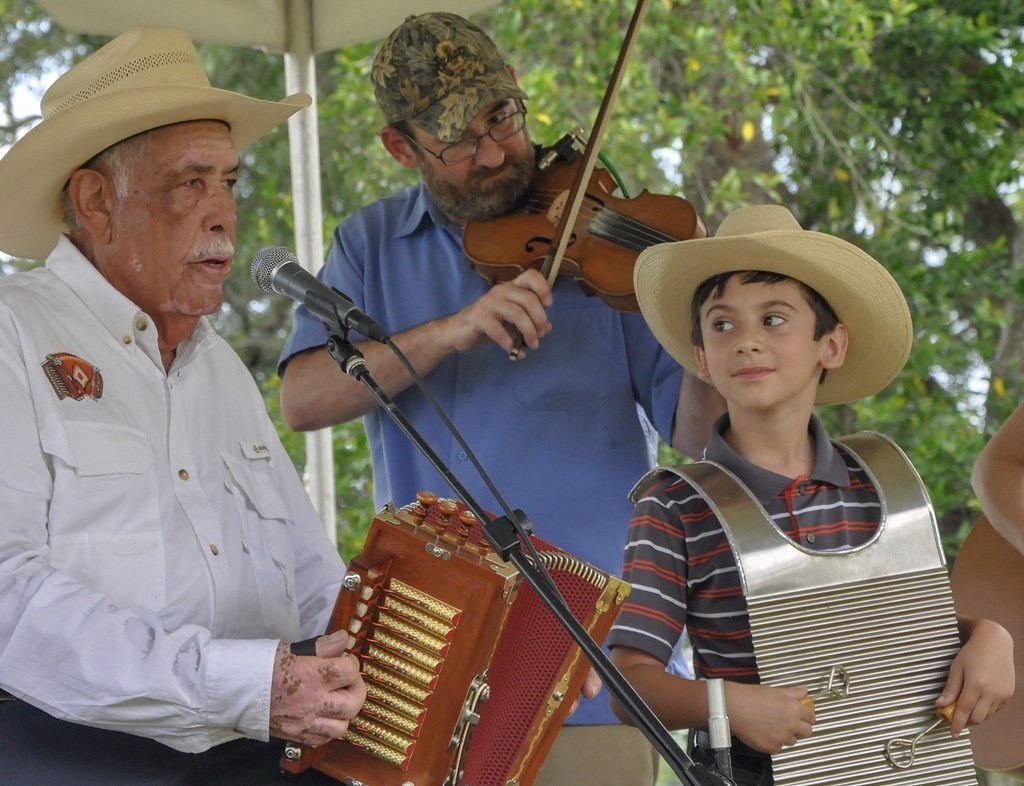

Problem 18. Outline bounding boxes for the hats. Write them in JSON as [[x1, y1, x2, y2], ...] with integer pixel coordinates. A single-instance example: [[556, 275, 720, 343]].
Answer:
[[370, 11, 531, 143], [0, 28, 312, 260], [633, 204, 913, 405]]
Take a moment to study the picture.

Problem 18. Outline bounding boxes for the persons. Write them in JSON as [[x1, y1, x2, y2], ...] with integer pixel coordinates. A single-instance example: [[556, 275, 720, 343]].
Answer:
[[969, 401, 1023, 556], [602, 203, 1014, 786], [0, 26, 369, 785], [278, 9, 726, 786]]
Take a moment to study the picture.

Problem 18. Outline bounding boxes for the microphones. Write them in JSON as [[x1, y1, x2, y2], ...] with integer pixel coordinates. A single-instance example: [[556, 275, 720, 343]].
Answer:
[[252, 245, 393, 347]]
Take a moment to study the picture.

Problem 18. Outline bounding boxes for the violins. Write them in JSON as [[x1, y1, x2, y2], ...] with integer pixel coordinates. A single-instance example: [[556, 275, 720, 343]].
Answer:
[[463, 144, 714, 317]]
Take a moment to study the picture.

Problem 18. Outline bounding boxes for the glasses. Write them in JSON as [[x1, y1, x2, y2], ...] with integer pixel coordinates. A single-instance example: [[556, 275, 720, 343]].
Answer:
[[400, 98, 527, 168]]
